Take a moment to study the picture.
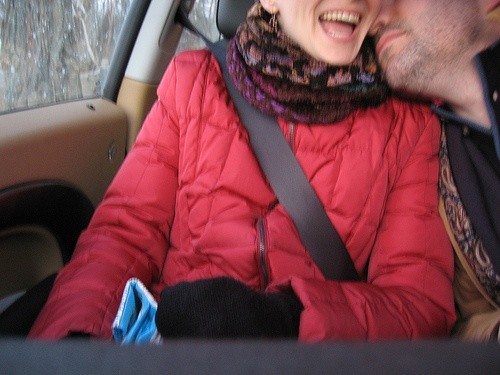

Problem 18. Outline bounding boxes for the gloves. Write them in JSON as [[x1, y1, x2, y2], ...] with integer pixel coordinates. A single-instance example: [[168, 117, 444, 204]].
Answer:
[[154, 275, 303, 339]]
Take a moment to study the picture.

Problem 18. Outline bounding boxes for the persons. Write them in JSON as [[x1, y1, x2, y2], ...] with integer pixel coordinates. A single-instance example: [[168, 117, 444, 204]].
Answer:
[[369, 0, 500, 340], [27, 0, 458, 339]]
